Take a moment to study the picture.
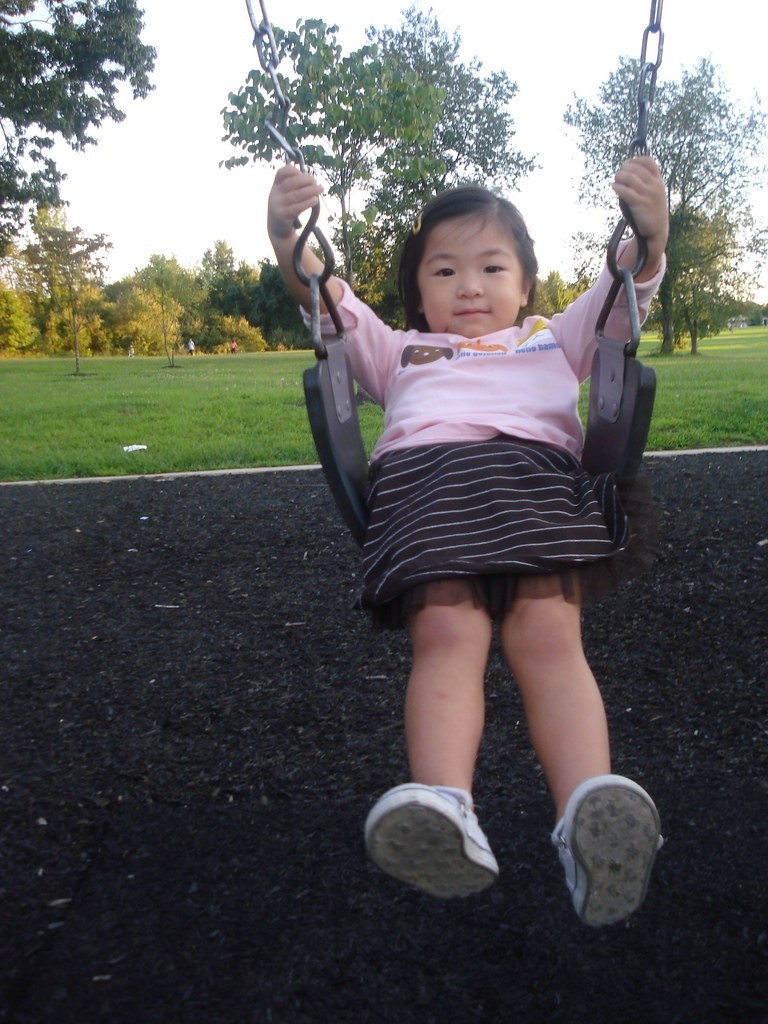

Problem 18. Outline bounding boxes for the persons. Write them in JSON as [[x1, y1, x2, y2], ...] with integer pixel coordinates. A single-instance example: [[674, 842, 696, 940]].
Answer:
[[188, 338, 194, 356], [230, 340, 237, 354], [128, 341, 134, 357], [267, 156, 669, 927]]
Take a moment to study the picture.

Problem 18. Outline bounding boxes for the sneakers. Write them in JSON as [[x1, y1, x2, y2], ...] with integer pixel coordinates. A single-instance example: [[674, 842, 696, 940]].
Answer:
[[363, 783, 499, 899], [550, 774, 663, 927]]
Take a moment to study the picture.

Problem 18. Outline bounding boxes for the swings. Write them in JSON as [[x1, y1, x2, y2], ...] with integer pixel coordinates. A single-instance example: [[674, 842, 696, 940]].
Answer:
[[236, 0, 674, 551]]
